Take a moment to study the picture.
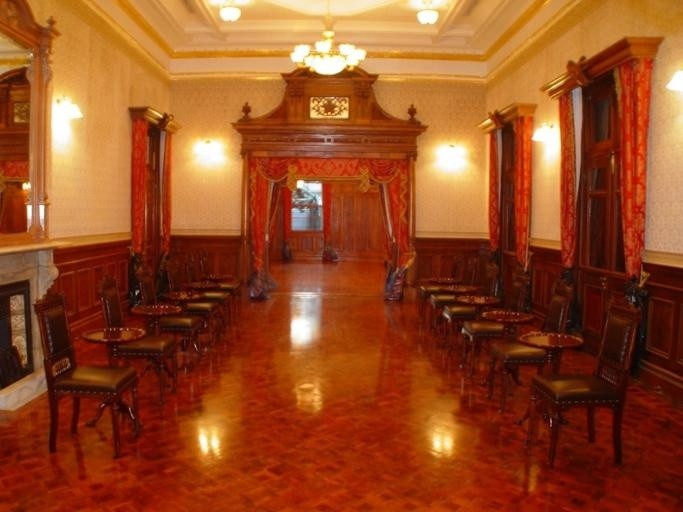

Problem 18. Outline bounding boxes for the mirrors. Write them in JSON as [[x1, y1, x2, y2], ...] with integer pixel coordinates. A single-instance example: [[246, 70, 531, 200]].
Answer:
[[0, 0, 61, 247]]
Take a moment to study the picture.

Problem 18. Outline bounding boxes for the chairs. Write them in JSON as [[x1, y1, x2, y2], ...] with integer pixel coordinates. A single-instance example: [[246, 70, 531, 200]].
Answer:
[[136, 248, 240, 372], [96, 275, 179, 404], [416, 252, 536, 379], [36, 289, 141, 457], [485, 263, 578, 412], [526, 300, 643, 468]]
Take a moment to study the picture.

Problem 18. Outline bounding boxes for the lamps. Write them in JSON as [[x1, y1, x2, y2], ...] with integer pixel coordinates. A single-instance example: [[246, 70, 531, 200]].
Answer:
[[289, 10, 366, 76], [412, 0, 445, 25], [210, 0, 247, 22]]
